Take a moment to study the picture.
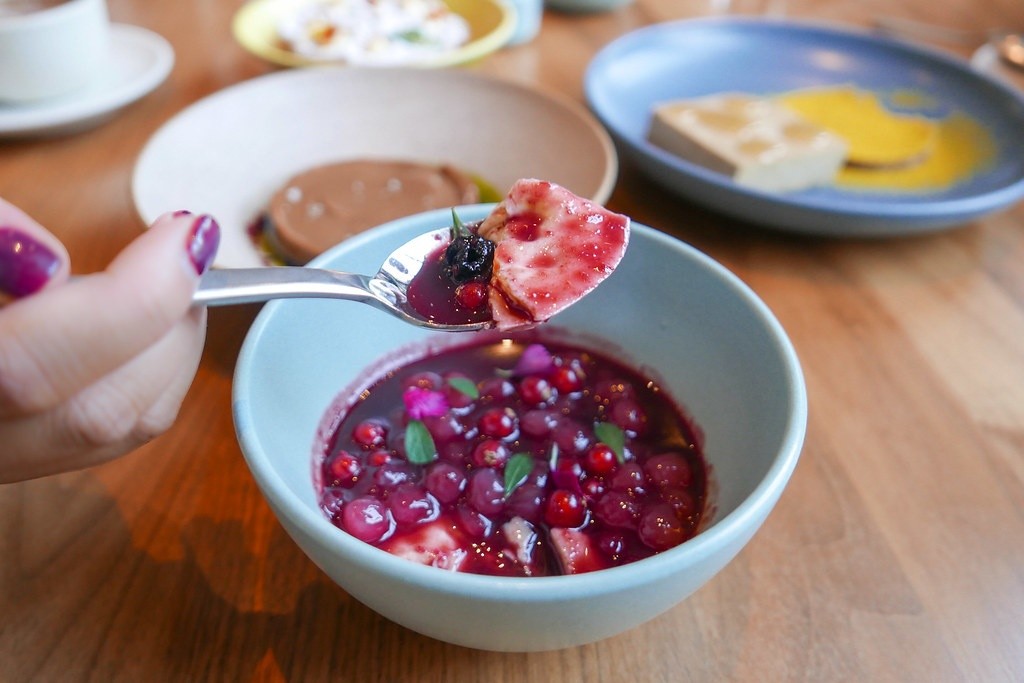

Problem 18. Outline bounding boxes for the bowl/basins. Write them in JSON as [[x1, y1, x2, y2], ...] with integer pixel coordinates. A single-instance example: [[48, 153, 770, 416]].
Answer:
[[230, 202, 809, 654]]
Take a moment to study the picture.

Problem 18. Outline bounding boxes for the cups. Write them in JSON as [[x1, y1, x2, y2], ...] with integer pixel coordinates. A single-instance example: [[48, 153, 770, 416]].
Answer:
[[0, 0, 115, 103], [543, 0, 619, 15], [503, 0, 543, 48]]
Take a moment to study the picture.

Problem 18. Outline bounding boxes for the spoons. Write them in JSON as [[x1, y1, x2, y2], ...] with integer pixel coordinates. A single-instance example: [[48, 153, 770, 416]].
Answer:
[[57, 215, 499, 335]]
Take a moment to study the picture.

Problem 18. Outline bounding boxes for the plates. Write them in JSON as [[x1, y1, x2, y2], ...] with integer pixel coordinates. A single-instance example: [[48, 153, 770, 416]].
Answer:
[[229, 0, 518, 72], [969, 36, 1024, 93], [129, 65, 621, 276], [582, 15, 1024, 242], [0, 21, 175, 142]]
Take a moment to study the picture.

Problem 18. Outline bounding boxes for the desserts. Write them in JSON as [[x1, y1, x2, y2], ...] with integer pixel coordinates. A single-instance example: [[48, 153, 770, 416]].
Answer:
[[649, 90, 845, 193], [266, 157, 478, 266]]
